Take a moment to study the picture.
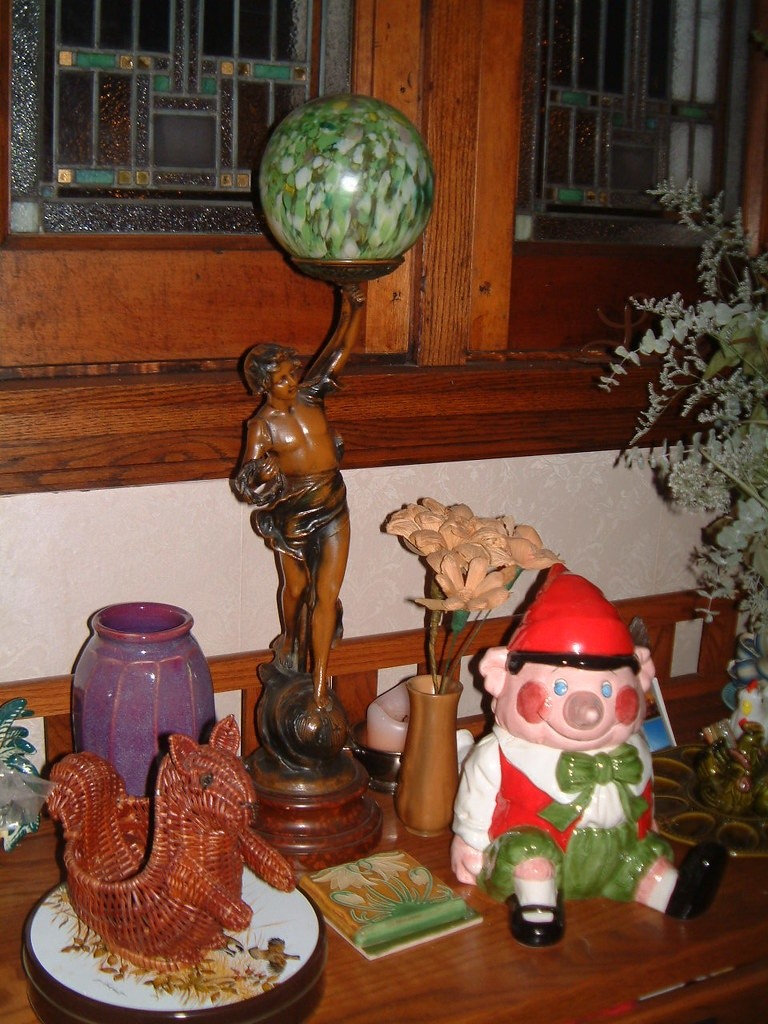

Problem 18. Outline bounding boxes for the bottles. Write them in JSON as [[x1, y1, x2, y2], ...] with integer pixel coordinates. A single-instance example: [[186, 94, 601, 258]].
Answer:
[[71, 602, 213, 796]]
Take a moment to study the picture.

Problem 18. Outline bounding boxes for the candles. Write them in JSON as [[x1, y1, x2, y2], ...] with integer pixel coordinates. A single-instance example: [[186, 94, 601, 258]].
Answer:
[[346, 679, 412, 792]]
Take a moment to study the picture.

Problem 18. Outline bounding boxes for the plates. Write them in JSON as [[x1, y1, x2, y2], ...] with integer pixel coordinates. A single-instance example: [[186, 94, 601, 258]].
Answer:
[[649, 741, 768, 860]]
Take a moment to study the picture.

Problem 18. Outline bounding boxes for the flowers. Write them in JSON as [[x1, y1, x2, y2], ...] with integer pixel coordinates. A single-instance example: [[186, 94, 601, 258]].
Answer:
[[375, 492, 567, 692], [600, 175, 768, 627]]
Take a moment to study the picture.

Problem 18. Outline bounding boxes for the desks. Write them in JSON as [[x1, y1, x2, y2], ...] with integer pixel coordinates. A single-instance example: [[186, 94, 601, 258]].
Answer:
[[0, 699, 768, 1024]]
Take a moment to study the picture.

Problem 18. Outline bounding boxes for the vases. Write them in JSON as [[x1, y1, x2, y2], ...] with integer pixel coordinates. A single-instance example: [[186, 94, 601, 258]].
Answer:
[[396, 676, 464, 838]]
